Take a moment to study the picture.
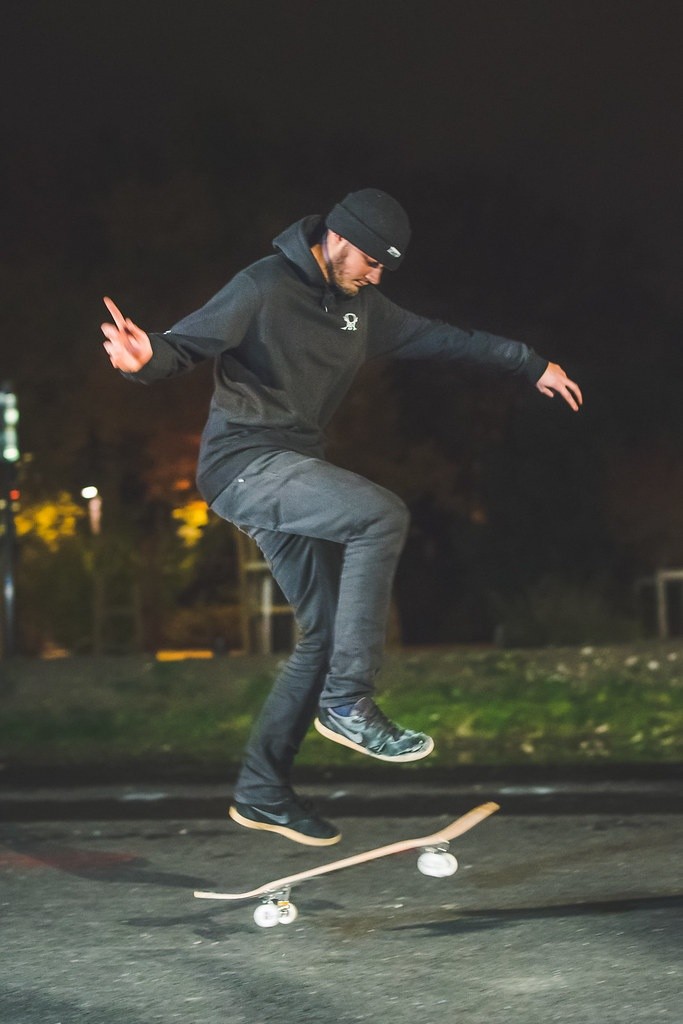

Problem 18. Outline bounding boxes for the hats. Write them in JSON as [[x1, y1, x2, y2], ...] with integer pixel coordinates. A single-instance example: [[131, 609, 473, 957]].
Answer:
[[327, 187, 412, 273]]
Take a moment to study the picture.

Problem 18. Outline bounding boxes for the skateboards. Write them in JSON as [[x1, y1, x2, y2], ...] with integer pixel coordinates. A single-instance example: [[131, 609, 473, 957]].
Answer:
[[192, 799, 501, 929]]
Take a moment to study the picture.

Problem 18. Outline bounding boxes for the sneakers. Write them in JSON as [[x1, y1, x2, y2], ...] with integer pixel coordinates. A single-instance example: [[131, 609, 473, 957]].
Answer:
[[228, 791, 342, 847], [314, 696, 435, 762]]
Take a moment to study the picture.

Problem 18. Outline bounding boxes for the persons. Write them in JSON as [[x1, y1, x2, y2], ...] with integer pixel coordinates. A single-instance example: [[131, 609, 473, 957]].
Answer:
[[100, 189, 582, 847]]
